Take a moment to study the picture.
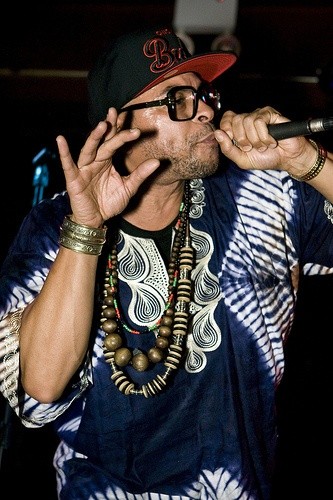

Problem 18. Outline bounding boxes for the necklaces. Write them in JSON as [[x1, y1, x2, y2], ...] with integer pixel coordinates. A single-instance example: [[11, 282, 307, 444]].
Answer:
[[100, 180, 194, 399]]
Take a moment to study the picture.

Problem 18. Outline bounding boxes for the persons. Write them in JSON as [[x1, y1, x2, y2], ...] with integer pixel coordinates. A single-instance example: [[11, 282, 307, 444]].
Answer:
[[1, 25, 333, 500]]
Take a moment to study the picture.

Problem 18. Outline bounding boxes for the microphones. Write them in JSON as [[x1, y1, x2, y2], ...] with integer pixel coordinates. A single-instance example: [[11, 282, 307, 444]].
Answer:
[[229, 116, 333, 150]]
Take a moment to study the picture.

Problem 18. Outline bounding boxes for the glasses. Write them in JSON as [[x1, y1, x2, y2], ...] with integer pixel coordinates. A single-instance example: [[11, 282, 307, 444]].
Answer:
[[120, 80, 219, 122]]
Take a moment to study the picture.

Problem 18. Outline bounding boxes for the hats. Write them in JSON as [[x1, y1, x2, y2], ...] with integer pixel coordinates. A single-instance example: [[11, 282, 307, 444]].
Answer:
[[86, 27, 239, 130]]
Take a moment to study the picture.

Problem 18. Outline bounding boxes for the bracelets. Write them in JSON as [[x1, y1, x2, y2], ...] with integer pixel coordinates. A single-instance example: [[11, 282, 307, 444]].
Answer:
[[287, 138, 328, 182], [58, 215, 107, 256]]
